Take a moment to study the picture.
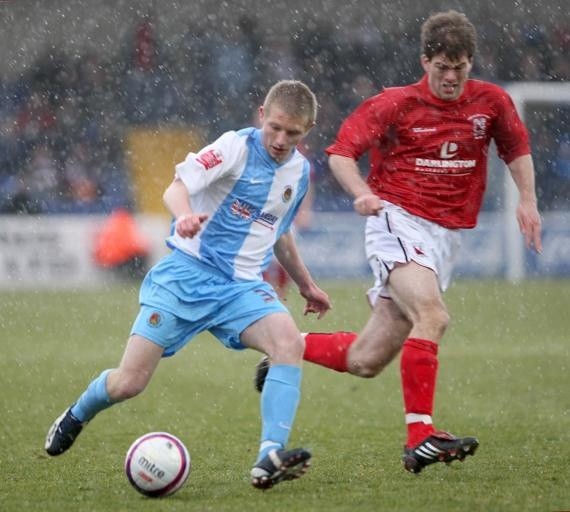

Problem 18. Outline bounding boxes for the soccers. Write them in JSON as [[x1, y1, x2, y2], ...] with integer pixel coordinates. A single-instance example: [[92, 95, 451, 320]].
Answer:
[[125, 432, 191, 500]]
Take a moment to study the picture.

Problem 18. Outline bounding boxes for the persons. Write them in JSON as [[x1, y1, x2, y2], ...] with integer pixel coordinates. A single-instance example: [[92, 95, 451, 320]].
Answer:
[[42, 79, 333, 488], [251, 8, 544, 476]]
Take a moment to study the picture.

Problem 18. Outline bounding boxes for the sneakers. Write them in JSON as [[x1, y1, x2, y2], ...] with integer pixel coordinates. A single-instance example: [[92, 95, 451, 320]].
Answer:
[[405, 434, 480, 473], [255, 356, 272, 393], [45, 402, 94, 456], [250, 448, 311, 489]]
